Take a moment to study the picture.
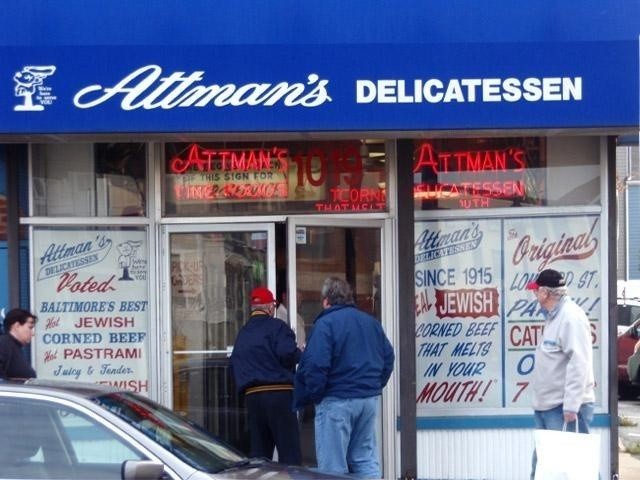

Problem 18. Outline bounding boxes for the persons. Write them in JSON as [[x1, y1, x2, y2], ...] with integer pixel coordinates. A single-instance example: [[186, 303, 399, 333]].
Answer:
[[525, 269, 604, 480], [292, 276, 395, 480], [1, 307, 39, 379], [226, 286, 306, 467], [273, 281, 307, 370]]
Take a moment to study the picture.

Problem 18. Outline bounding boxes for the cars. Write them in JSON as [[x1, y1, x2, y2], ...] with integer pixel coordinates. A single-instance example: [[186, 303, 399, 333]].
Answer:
[[0, 378, 373, 479], [173, 357, 319, 466], [616, 316, 639, 398]]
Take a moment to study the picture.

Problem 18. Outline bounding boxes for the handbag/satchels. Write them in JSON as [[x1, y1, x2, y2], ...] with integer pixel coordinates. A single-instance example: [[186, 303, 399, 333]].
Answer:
[[533, 415, 601, 480]]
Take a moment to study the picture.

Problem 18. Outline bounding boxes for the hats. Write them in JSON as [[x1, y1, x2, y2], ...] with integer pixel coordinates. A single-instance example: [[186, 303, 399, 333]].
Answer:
[[526, 270, 566, 289], [251, 288, 278, 305]]
